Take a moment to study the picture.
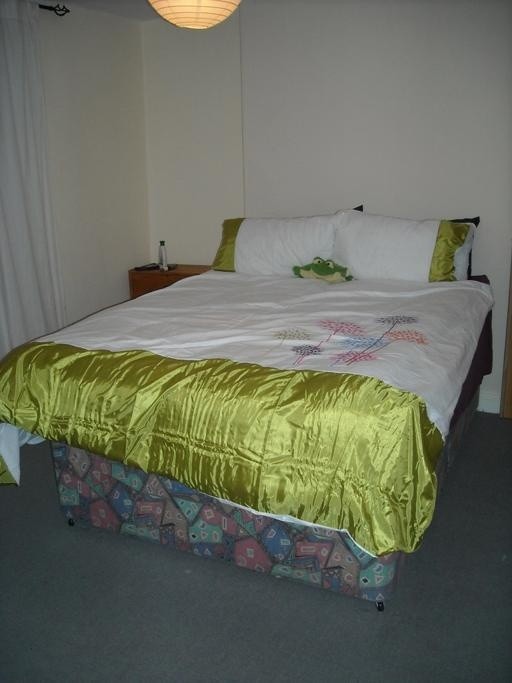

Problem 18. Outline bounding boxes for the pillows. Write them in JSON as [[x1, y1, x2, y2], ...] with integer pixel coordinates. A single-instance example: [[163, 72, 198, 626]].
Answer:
[[212, 211, 341, 276], [334, 211, 476, 285]]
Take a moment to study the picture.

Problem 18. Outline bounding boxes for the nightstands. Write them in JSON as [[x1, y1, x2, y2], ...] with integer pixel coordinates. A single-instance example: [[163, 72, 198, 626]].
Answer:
[[128, 264, 211, 296]]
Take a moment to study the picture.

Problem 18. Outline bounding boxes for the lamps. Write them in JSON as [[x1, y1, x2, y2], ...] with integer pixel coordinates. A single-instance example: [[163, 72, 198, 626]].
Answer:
[[148, 0, 241, 29]]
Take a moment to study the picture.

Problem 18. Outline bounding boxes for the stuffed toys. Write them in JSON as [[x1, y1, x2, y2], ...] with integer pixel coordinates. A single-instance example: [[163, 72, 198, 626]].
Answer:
[[291, 256, 354, 282]]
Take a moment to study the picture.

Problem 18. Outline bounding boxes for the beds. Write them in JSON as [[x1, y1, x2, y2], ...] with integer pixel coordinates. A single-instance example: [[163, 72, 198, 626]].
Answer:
[[0, 206, 494, 614]]
